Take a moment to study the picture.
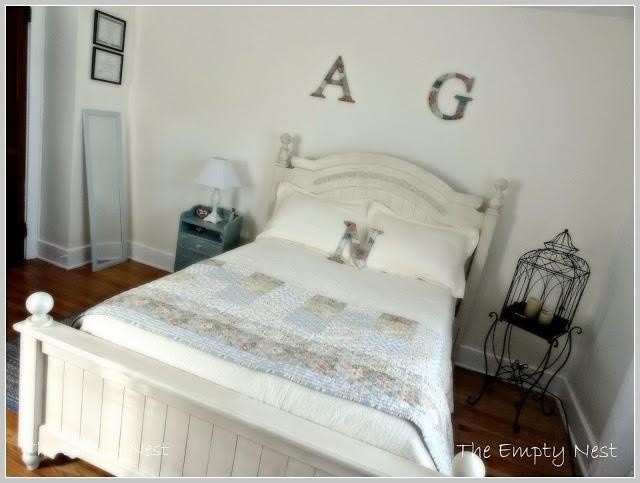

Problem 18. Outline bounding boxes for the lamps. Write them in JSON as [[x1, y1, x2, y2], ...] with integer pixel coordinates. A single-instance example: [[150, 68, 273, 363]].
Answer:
[[193, 157, 243, 223]]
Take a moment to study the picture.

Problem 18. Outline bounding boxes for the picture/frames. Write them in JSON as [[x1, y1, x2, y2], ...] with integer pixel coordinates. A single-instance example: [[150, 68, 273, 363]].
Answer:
[[90, 9, 127, 85]]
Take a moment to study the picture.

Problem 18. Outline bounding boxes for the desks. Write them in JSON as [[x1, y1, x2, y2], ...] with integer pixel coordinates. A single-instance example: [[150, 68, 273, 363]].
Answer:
[[466, 301, 570, 434]]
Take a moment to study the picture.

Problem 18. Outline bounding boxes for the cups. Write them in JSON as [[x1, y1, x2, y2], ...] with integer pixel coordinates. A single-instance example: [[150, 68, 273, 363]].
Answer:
[[524, 297, 554, 325]]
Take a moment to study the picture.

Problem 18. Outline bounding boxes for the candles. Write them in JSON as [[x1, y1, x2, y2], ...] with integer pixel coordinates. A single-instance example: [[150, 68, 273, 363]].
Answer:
[[525, 295, 554, 325]]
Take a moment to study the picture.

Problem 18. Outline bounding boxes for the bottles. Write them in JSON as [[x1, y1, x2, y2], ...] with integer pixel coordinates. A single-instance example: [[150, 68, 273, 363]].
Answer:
[[228, 207, 236, 219]]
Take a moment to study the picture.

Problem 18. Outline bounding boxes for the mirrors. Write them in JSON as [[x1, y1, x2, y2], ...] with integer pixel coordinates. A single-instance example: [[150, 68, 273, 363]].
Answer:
[[82, 110, 128, 274]]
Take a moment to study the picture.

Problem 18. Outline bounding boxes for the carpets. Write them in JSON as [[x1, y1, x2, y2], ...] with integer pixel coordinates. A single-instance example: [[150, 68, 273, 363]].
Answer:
[[6, 303, 91, 413]]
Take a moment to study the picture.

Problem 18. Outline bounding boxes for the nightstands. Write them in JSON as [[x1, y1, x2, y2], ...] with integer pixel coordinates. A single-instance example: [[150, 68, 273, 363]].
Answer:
[[174, 204, 243, 273]]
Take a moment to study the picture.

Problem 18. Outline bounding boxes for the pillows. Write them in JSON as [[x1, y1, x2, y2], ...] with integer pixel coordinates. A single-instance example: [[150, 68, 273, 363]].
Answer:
[[253, 181, 482, 302]]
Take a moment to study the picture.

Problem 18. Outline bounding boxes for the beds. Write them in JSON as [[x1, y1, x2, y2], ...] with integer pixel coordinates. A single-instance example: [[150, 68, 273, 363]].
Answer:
[[10, 131, 508, 478]]
